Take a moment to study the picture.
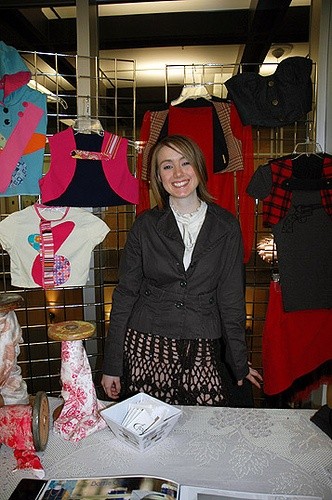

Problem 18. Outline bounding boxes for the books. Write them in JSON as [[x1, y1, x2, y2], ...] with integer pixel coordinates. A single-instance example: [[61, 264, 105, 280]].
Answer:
[[33, 474, 317, 500]]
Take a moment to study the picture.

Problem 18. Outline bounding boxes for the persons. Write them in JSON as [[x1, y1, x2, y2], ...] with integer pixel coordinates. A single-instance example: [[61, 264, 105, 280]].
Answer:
[[98, 137, 265, 408]]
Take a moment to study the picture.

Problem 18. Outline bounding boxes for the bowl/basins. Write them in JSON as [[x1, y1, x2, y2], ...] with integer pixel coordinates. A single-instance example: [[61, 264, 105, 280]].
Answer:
[[99, 392, 182, 452]]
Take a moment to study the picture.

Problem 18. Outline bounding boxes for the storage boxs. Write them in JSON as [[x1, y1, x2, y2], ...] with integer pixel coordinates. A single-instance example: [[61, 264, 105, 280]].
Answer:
[[100, 392, 182, 452]]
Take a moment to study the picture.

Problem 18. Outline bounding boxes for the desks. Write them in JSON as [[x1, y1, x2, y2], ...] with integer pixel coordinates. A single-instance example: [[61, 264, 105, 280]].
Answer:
[[1, 398, 331, 500]]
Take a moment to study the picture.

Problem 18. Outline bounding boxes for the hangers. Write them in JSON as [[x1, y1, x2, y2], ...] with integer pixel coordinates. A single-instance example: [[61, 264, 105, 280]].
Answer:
[[72, 95, 107, 139], [171, 64, 214, 107], [287, 118, 324, 163]]
